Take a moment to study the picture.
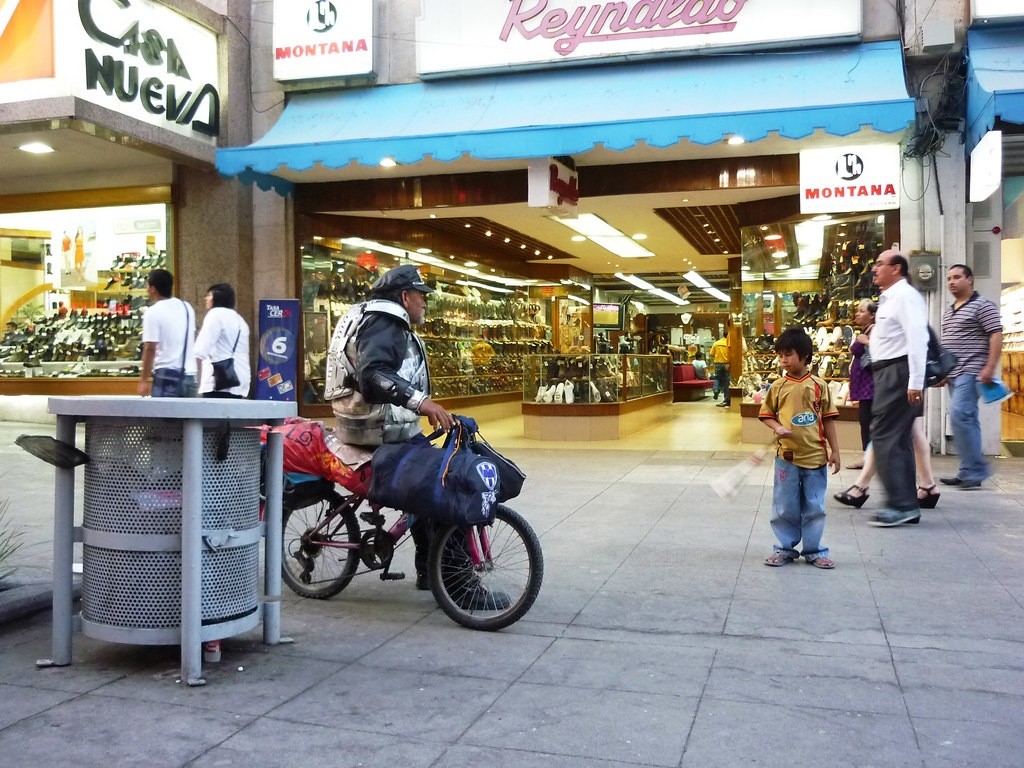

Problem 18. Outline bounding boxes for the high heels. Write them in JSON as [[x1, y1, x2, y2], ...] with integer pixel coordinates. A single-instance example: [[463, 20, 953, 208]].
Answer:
[[833, 485, 869, 509], [917, 484, 941, 509]]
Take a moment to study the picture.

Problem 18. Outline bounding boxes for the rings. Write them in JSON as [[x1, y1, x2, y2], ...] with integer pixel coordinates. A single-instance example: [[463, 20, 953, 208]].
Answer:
[[915, 397, 919, 400]]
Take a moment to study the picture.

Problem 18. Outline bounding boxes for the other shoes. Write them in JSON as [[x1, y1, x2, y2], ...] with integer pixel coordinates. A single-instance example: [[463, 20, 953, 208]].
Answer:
[[0, 251, 168, 377], [535, 357, 617, 403], [940, 476, 982, 486], [744, 239, 880, 406], [869, 507, 921, 526], [716, 402, 730, 407], [714, 390, 718, 399], [306, 276, 557, 403]]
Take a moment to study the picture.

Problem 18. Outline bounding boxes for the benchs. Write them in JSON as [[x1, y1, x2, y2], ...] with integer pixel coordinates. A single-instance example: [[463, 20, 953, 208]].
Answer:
[[670, 361, 714, 400]]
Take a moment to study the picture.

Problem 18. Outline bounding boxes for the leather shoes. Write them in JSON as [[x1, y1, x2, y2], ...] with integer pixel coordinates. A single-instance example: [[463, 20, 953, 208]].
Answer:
[[416, 574, 480, 590], [449, 585, 510, 610]]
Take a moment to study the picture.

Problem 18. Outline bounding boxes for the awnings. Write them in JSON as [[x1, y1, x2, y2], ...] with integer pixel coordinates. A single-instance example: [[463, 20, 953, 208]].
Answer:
[[213, 41, 916, 193]]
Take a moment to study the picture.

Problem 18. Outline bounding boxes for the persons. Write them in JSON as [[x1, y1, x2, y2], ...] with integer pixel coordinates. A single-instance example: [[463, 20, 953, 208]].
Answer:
[[709, 320, 731, 407], [138, 269, 196, 397], [74, 226, 84, 281], [832, 324, 941, 509], [692, 352, 715, 380], [869, 249, 930, 527], [3, 321, 17, 339], [844, 297, 877, 468], [941, 265, 1002, 487], [61, 230, 72, 275], [195, 283, 251, 398], [758, 329, 841, 568], [322, 264, 510, 610]]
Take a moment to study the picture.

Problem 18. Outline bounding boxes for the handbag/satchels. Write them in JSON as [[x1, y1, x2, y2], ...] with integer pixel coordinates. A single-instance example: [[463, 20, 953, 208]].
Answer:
[[211, 358, 240, 391], [151, 368, 194, 397], [452, 439, 526, 504], [924, 325, 957, 387], [368, 424, 500, 526]]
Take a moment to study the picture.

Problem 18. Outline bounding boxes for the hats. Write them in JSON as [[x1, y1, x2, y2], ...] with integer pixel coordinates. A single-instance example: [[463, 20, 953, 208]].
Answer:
[[371, 265, 433, 299]]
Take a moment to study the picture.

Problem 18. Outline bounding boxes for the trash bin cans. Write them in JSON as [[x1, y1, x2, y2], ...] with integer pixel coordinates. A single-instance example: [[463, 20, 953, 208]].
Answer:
[[36, 395, 297, 685]]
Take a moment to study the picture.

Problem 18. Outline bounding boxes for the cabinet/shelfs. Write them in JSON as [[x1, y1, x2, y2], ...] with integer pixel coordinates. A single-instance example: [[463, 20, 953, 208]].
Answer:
[[743, 351, 858, 404], [98, 261, 164, 299], [418, 336, 554, 399]]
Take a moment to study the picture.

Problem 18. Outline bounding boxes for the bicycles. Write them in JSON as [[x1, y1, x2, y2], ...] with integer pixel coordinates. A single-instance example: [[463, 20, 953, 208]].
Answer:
[[261, 413, 545, 633]]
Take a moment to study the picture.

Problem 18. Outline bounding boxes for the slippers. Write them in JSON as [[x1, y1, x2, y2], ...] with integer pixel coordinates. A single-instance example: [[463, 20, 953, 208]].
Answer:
[[764, 552, 792, 566], [812, 557, 835, 568]]
